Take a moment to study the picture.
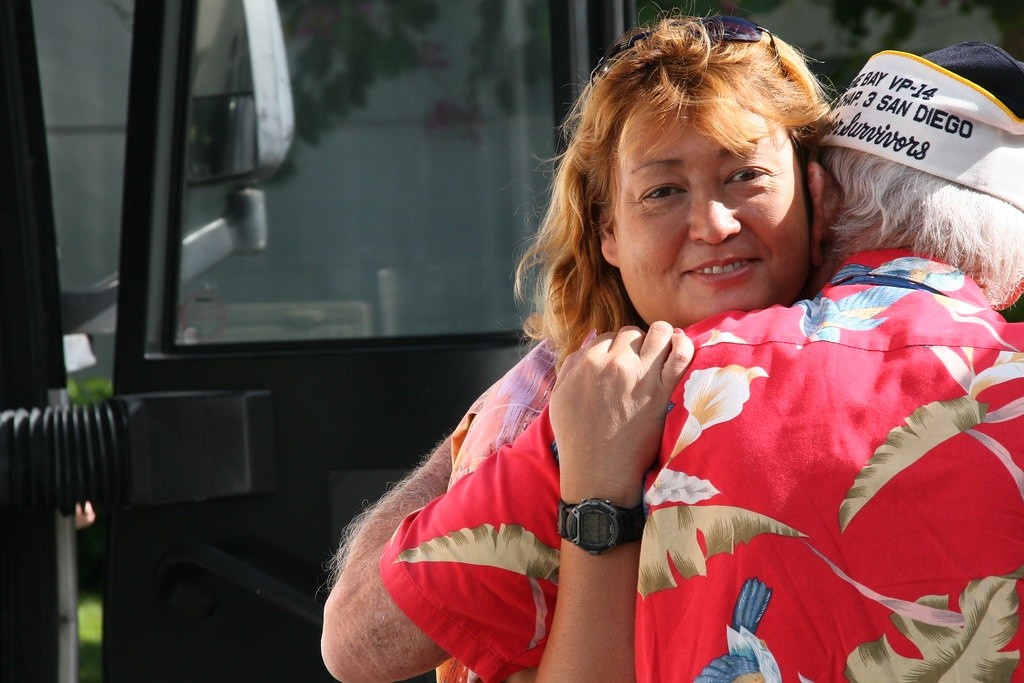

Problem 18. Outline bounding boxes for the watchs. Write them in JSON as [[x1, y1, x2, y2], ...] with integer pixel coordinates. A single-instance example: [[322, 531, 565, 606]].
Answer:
[[558, 497, 645, 555]]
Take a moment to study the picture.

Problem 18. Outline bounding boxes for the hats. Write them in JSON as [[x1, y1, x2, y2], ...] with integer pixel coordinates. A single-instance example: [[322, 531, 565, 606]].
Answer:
[[817, 39, 1024, 211]]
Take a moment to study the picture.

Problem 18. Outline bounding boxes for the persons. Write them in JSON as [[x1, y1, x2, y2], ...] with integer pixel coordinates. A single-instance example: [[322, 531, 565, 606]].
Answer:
[[321, 15, 1024, 683]]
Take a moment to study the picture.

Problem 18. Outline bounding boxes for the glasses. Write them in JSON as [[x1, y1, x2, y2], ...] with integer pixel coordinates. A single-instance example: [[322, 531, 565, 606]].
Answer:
[[590, 15, 789, 88]]
